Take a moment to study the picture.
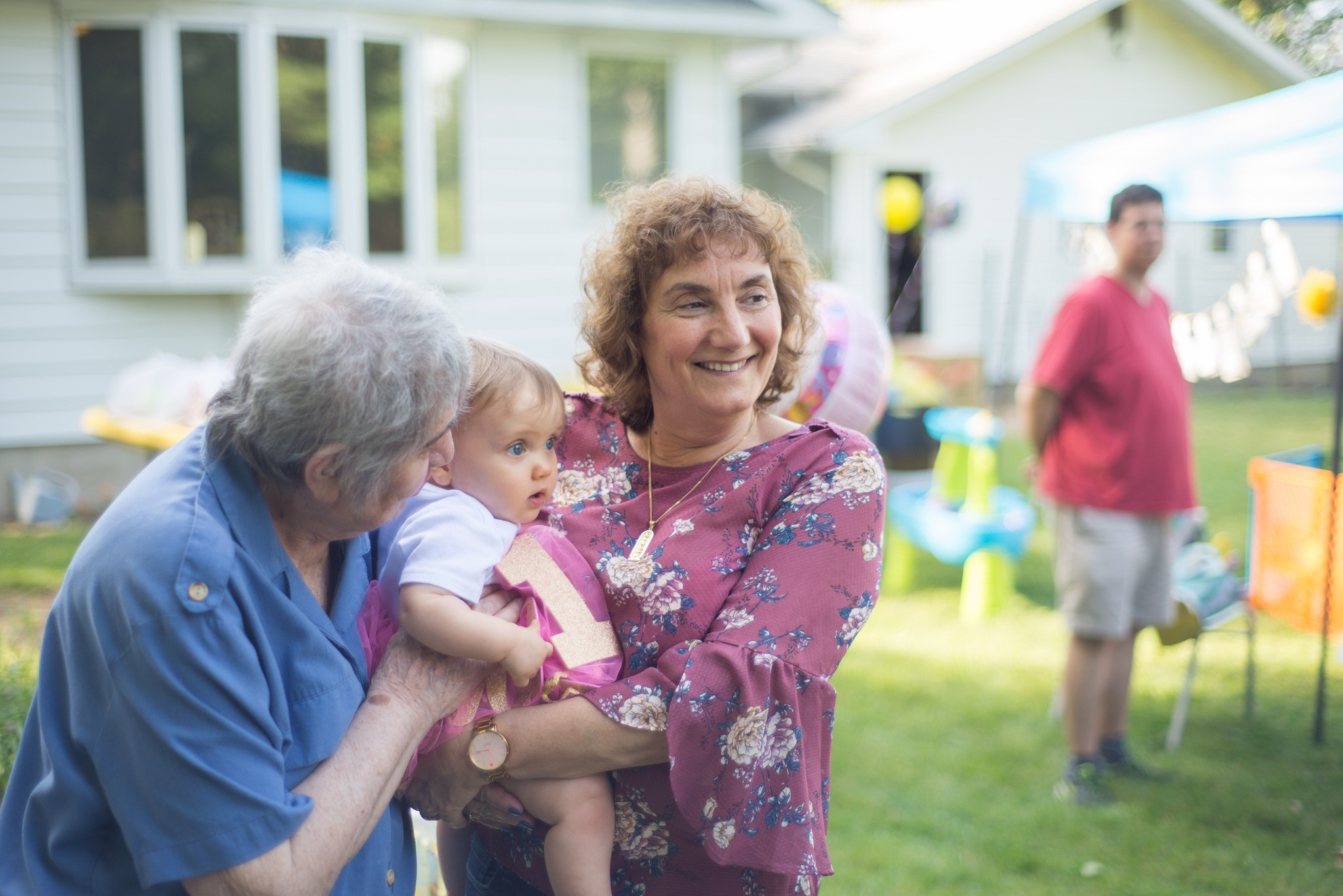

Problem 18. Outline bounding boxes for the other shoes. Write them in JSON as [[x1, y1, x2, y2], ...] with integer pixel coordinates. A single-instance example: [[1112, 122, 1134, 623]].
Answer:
[[1103, 749, 1179, 783], [1053, 772, 1112, 807]]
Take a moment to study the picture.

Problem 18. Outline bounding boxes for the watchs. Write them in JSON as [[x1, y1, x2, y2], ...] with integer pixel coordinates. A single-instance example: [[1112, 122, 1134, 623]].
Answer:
[[464, 713, 510, 781]]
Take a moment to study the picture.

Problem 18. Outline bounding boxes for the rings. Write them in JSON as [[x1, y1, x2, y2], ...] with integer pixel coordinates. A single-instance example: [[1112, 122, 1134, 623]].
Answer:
[[469, 811, 474, 821]]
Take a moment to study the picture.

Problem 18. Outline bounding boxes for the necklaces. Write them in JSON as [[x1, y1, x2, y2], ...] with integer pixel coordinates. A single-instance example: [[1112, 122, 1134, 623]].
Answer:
[[628, 405, 760, 563]]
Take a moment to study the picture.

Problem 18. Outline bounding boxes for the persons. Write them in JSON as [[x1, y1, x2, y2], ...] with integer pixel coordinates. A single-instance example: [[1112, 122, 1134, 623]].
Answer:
[[0, 161, 892, 896], [1016, 184, 1189, 810]]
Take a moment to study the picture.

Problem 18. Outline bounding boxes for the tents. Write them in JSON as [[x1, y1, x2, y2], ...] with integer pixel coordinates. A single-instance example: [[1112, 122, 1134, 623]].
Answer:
[[989, 69, 1343, 388]]
